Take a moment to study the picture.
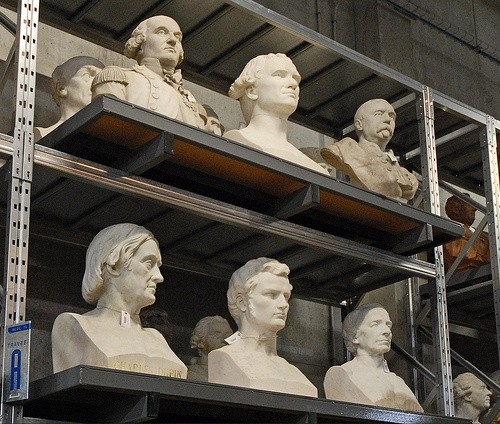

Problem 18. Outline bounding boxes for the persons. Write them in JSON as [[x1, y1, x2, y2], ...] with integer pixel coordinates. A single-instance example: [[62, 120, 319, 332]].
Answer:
[[186, 314, 234, 383], [201, 104, 225, 137], [323, 302, 426, 414], [32, 55, 106, 143], [89, 14, 208, 131], [320, 97, 419, 205], [207, 256, 320, 399], [52, 223, 189, 383], [452, 372, 493, 424], [298, 146, 332, 177], [222, 52, 332, 177], [440, 192, 490, 273]]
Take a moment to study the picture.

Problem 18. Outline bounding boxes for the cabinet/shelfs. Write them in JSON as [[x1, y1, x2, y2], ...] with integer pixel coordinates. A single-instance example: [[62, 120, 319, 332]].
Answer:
[[12, 0, 471, 424]]
